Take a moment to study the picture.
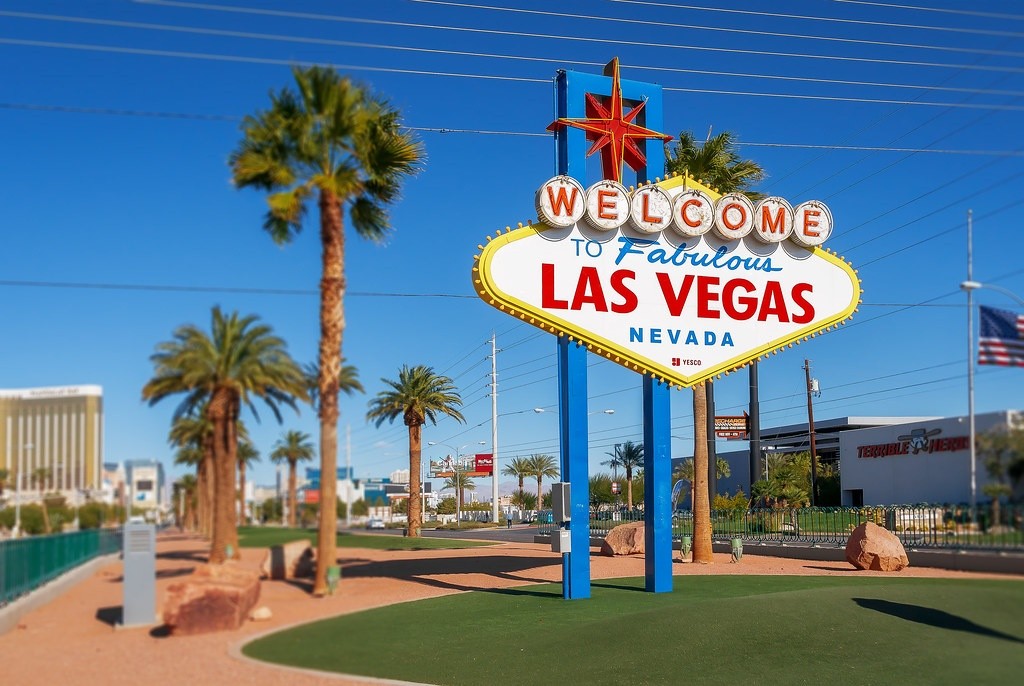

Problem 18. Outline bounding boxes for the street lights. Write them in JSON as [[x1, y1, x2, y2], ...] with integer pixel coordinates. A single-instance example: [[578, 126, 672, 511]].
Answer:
[[427, 441, 486, 526]]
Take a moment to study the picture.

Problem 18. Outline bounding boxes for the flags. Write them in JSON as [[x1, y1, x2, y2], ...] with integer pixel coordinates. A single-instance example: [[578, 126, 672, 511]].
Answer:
[[978, 304, 1024, 368]]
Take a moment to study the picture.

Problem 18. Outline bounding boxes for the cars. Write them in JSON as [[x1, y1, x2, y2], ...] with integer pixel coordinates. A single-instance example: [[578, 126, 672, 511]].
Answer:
[[365, 519, 386, 530]]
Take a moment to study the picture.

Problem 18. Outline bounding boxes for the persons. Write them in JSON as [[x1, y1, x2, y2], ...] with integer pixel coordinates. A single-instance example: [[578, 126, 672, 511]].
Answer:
[[507, 511, 513, 529]]
